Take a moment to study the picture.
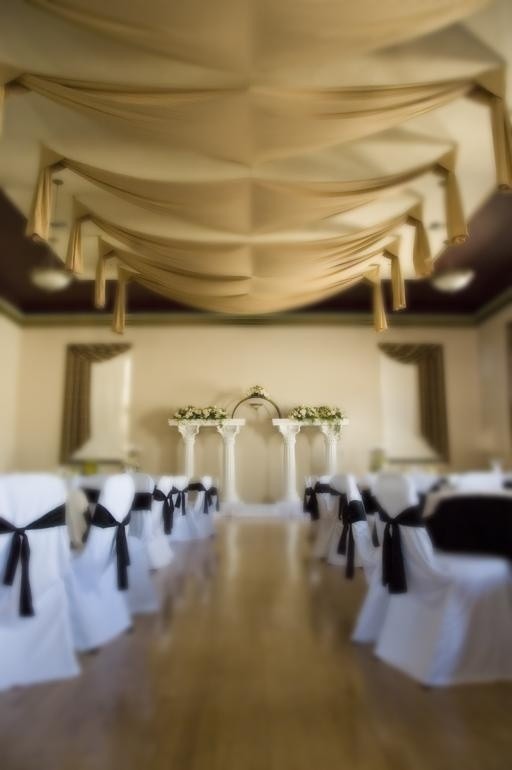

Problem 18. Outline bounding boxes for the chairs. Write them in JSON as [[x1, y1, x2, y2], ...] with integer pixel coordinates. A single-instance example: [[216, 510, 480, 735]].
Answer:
[[305, 458, 512, 688], [1, 473, 220, 694]]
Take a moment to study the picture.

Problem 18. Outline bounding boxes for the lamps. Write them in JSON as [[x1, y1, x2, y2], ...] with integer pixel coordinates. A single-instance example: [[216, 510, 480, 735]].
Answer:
[[432, 242, 476, 296], [29, 241, 73, 296]]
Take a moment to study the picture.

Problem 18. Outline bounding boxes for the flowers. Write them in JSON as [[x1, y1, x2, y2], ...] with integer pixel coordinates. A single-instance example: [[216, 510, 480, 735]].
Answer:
[[286, 404, 346, 434], [172, 403, 229, 431], [245, 382, 269, 400]]
